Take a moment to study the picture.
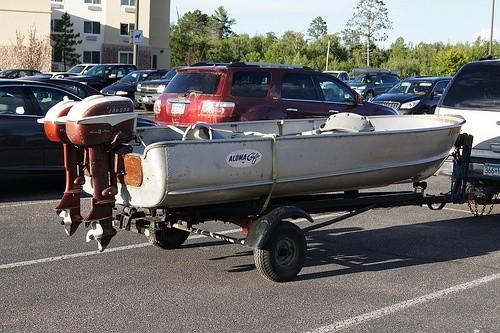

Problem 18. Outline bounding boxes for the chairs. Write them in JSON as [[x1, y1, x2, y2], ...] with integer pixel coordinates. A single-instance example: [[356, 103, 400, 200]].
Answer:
[[0, 95, 27, 115]]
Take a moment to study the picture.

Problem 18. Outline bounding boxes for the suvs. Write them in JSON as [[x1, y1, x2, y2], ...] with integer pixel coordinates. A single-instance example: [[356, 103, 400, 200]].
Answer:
[[430, 56, 500, 205], [153, 60, 402, 127], [363, 75, 454, 118]]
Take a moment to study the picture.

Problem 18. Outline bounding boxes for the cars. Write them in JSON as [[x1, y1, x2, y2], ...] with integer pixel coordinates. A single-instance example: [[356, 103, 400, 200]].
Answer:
[[0, 60, 404, 102], [0, 78, 161, 196]]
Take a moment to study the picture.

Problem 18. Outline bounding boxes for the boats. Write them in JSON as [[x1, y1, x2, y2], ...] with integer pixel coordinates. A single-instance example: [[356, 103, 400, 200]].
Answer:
[[44, 115, 465, 253]]
